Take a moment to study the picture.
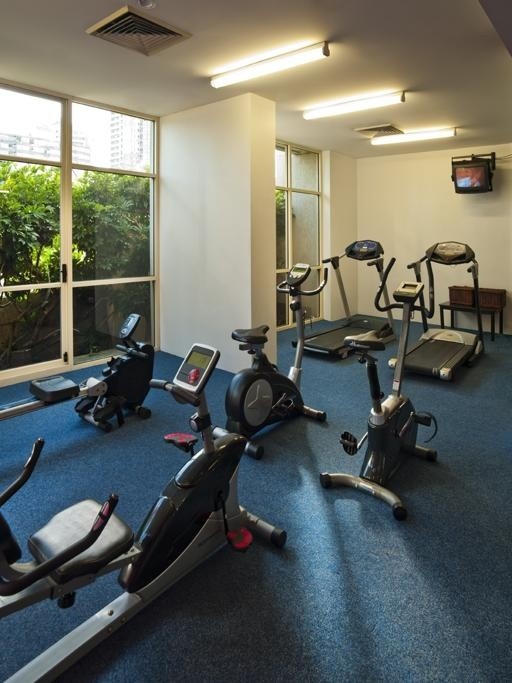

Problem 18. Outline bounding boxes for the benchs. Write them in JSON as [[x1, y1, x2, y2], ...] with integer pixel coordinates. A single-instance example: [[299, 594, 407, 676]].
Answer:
[[439, 300, 504, 341]]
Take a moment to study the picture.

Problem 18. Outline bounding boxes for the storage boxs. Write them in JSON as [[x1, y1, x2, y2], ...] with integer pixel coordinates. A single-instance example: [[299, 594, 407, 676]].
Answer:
[[448, 285, 506, 308]]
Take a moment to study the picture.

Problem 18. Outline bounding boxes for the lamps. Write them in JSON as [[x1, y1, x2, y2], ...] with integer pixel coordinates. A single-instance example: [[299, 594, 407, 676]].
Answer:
[[210, 41, 457, 146]]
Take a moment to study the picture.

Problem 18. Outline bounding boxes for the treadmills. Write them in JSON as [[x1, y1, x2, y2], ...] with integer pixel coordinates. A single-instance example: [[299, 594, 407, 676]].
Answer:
[[388, 241, 485, 381], [291, 240, 396, 359]]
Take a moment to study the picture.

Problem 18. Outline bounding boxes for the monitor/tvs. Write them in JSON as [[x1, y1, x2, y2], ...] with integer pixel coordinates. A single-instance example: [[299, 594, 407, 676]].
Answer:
[[451, 160, 493, 195]]
